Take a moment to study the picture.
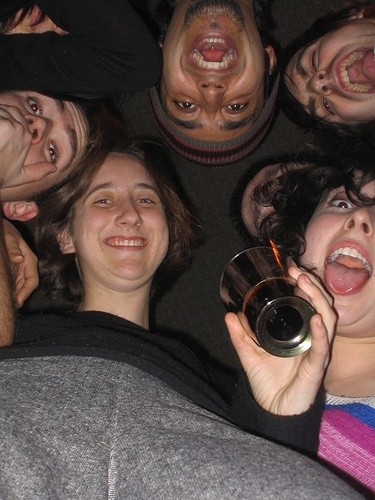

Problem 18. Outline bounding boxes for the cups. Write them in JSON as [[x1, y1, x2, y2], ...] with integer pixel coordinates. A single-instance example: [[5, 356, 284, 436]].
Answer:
[[220, 246, 317, 356]]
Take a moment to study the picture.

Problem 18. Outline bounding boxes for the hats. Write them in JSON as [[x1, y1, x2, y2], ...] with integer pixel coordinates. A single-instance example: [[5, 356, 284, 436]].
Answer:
[[146, 67, 283, 168]]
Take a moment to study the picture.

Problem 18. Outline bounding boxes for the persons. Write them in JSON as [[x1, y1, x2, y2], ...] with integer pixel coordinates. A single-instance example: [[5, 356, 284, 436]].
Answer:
[[0, 135, 368, 500], [0, 0, 375, 500]]
